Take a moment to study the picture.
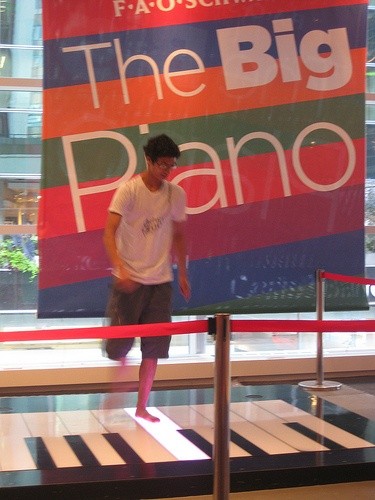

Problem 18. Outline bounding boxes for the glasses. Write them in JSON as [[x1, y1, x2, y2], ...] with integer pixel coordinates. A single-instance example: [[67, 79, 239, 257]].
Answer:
[[153, 161, 178, 169]]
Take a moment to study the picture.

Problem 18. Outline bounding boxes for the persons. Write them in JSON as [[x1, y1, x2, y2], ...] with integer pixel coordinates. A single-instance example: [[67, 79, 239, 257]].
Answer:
[[100, 134, 192, 421]]
